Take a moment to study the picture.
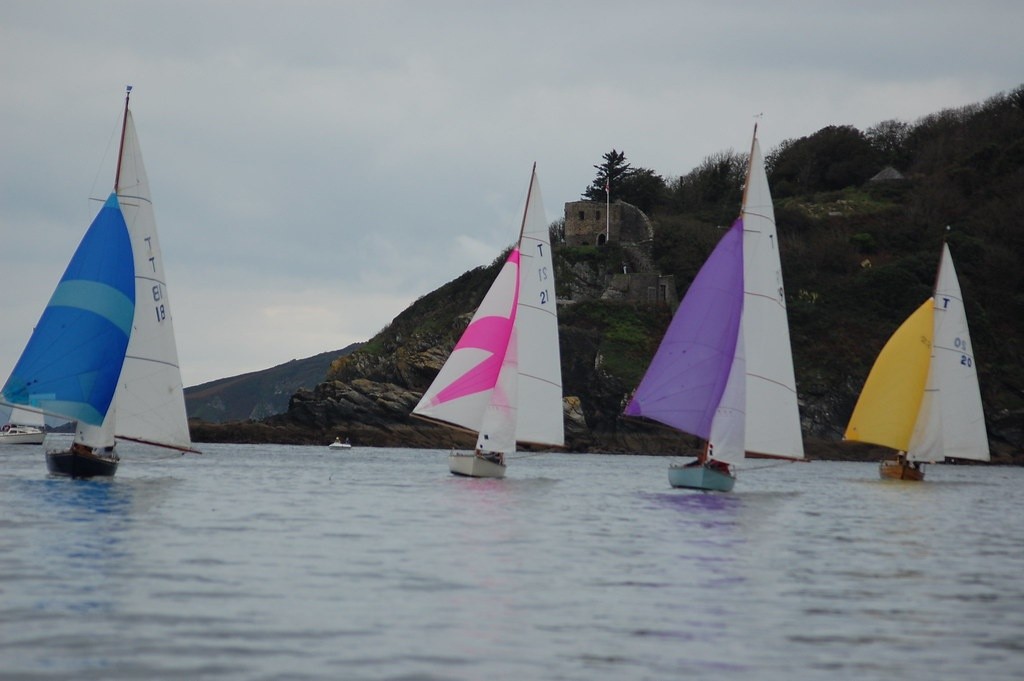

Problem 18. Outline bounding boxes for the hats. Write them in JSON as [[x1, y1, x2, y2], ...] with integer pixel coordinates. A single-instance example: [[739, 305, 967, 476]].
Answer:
[[336, 437, 339, 439]]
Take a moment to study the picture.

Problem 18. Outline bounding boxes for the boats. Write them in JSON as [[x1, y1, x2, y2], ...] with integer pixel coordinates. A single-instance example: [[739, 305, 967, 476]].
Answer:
[[329, 442, 352, 449]]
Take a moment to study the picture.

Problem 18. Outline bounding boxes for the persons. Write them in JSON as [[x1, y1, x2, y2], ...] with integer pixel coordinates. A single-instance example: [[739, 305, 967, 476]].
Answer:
[[477, 450, 503, 464], [335, 437, 341, 443], [11, 424, 17, 432], [344, 438, 350, 444], [70, 441, 120, 461], [684, 441, 730, 473], [897, 451, 920, 471]]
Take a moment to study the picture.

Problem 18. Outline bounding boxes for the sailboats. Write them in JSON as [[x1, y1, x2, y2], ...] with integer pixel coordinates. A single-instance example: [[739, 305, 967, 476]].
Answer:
[[621, 125, 811, 490], [1, 86, 200, 476], [411, 163, 562, 478], [1, 397, 46, 444], [843, 244, 990, 479]]
